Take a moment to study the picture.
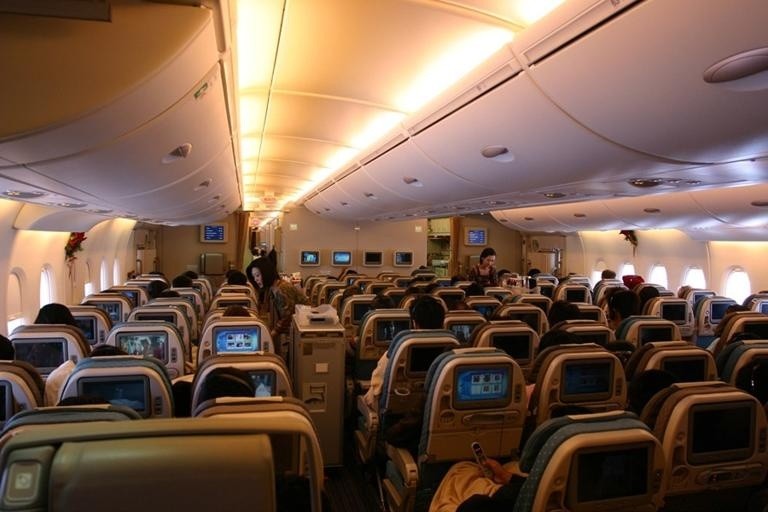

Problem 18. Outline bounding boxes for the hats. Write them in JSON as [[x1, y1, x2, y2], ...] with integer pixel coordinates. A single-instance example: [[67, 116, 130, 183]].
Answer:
[[623, 276, 644, 288]]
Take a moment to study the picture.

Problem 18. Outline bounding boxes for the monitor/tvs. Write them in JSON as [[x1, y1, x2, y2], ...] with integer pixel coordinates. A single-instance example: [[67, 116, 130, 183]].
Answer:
[[200, 222, 229, 243], [12, 272, 262, 375], [572, 330, 611, 346], [638, 324, 675, 348], [524, 300, 549, 315], [248, 369, 277, 398], [405, 343, 456, 378], [559, 357, 615, 404], [311, 271, 674, 311], [464, 227, 487, 245], [759, 302, 768, 313], [693, 292, 714, 311], [452, 362, 513, 411], [298, 249, 415, 267], [570, 441, 657, 507], [574, 308, 602, 322], [742, 320, 768, 342], [687, 402, 757, 466], [490, 331, 533, 366], [710, 301, 736, 324], [1, 383, 13, 421], [660, 301, 688, 325], [448, 321, 483, 346], [661, 355, 709, 384], [508, 310, 541, 336], [373, 317, 414, 348], [471, 303, 499, 322], [77, 375, 150, 418], [351, 301, 375, 325]]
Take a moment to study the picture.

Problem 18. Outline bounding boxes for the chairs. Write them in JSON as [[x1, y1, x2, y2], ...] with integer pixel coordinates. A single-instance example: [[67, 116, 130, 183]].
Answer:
[[1, 267, 767, 512]]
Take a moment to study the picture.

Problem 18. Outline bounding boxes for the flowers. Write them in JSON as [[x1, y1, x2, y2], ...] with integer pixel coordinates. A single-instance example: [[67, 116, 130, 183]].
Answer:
[[60, 230, 88, 279]]
